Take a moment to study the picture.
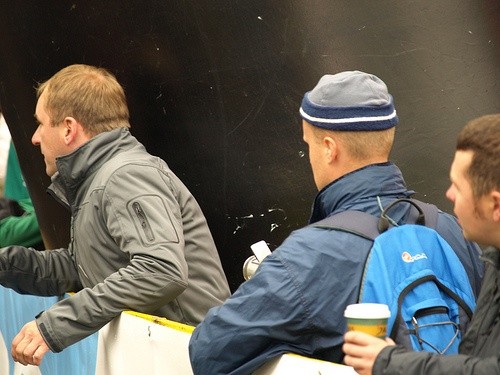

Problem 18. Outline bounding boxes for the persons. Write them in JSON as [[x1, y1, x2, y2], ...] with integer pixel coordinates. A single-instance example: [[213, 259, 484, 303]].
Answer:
[[341, 114, 500, 375], [196, 72, 484, 375], [0, 64, 234, 367]]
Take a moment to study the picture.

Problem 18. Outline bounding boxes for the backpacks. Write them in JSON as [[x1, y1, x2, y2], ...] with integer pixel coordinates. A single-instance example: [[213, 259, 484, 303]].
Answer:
[[306, 199, 477, 356]]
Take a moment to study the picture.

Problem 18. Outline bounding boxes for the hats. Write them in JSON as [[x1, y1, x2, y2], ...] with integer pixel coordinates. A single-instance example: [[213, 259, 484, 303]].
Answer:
[[298, 71, 398, 131]]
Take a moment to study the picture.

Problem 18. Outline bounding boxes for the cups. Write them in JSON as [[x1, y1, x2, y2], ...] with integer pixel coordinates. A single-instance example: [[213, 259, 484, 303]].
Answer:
[[343, 302, 392, 368]]
